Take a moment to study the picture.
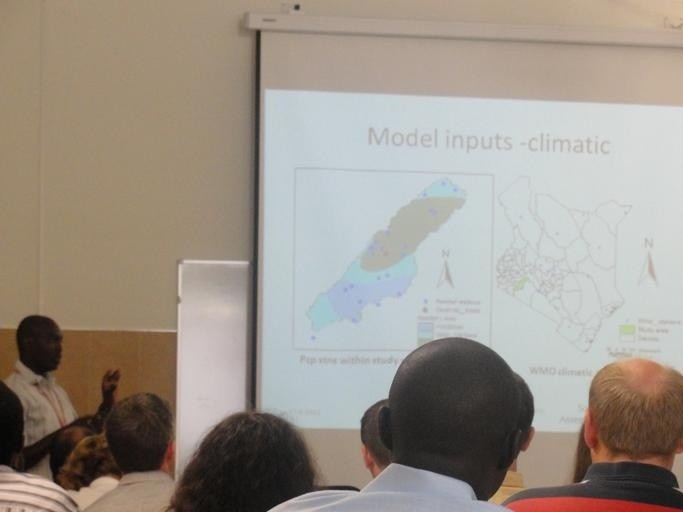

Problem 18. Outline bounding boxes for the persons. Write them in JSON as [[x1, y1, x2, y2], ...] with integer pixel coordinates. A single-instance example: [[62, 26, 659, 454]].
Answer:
[[499, 357, 682, 511], [1, 380, 79, 511], [0, 315, 122, 483], [167, 410, 319, 511], [269, 337, 535, 510], [49, 393, 176, 511]]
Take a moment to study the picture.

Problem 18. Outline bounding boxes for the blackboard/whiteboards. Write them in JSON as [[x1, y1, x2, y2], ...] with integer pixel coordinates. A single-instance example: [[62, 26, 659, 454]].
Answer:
[[175, 259, 256, 480]]
